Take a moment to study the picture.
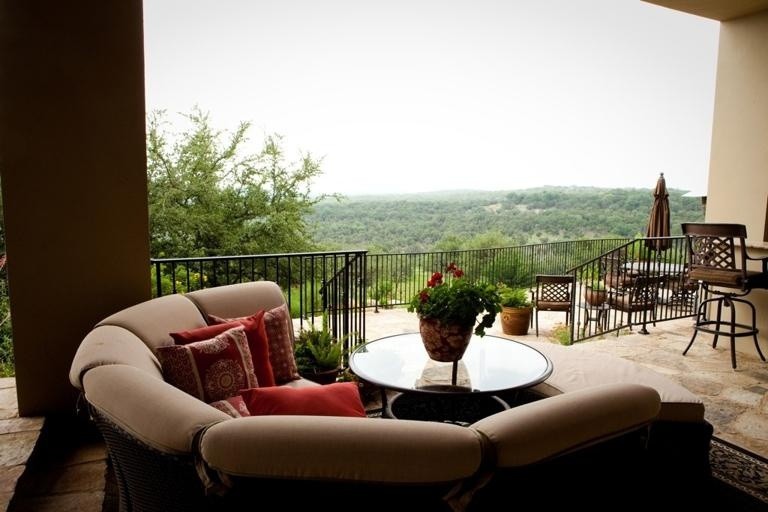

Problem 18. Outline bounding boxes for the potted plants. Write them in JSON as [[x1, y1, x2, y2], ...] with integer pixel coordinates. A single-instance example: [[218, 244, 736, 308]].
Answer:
[[499, 286, 533, 336]]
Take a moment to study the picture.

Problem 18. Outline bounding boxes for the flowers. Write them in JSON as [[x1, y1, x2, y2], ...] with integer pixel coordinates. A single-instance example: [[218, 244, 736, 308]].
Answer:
[[409, 263, 500, 333]]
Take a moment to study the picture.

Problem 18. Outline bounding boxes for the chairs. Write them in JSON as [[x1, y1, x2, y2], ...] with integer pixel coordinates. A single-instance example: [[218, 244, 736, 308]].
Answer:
[[535, 223, 768, 370]]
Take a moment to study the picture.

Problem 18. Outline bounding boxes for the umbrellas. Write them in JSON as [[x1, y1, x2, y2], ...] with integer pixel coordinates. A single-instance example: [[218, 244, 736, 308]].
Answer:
[[645, 173, 673, 251]]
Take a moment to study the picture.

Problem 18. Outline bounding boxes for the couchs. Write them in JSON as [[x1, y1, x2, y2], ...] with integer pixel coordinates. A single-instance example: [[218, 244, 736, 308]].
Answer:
[[68, 281, 711, 511]]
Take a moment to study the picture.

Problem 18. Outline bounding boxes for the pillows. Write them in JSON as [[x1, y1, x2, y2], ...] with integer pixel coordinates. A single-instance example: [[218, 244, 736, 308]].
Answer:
[[156, 302, 369, 417]]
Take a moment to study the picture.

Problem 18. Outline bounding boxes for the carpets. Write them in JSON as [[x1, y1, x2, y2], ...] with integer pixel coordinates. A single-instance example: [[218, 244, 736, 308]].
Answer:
[[99, 379, 767, 512]]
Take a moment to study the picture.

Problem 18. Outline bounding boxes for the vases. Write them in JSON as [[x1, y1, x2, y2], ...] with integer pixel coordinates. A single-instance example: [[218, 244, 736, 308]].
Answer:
[[419, 320, 473, 362]]
